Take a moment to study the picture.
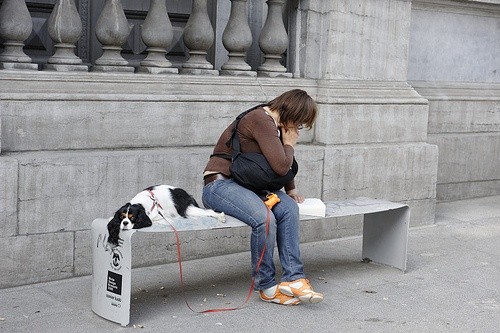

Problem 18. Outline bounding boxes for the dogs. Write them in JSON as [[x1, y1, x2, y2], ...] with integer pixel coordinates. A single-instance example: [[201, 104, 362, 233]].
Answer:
[[107, 184, 226, 245]]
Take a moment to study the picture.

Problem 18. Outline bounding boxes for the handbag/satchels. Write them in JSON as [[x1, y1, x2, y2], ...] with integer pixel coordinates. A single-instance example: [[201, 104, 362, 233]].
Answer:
[[210, 101, 297, 196]]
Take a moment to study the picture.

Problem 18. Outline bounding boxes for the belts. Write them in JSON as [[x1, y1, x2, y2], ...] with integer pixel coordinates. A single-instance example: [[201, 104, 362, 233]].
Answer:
[[204, 173, 227, 185]]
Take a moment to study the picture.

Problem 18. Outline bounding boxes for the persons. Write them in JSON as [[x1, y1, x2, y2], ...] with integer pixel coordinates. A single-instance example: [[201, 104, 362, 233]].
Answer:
[[202, 89, 325, 305]]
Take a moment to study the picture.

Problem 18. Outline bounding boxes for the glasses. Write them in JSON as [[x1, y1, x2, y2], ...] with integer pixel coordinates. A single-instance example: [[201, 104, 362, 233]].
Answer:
[[297, 123, 303, 129]]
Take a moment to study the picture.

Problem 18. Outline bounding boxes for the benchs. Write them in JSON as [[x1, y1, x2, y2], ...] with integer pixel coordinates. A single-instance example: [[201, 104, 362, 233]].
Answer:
[[90, 196, 411, 326]]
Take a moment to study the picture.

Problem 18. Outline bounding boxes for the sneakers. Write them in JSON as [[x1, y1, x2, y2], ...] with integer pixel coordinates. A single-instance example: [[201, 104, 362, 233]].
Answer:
[[259, 284, 304, 305], [279, 278, 324, 303]]
